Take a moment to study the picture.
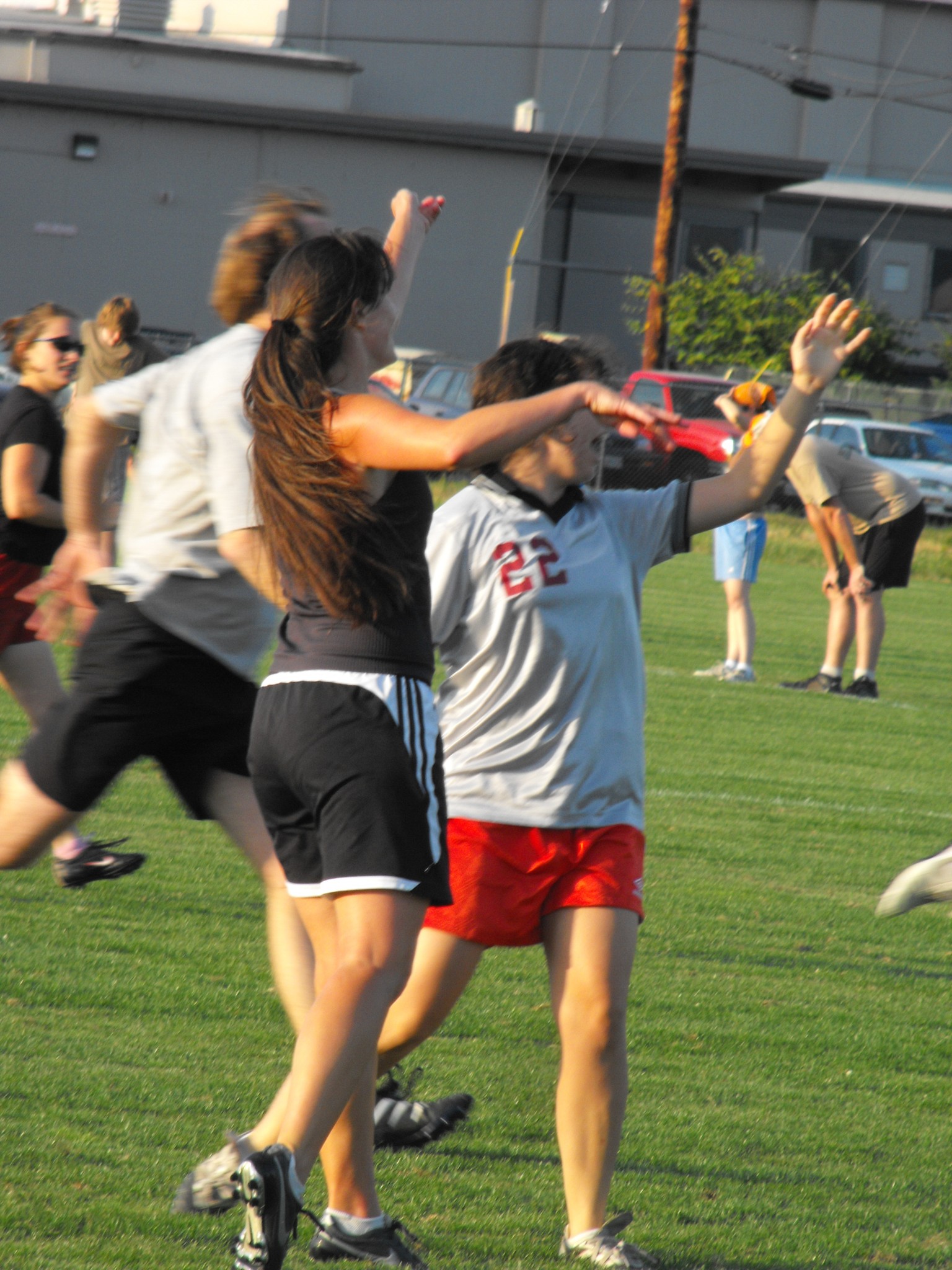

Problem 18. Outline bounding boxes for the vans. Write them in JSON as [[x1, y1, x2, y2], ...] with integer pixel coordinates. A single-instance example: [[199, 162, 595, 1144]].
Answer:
[[402, 363, 483, 420]]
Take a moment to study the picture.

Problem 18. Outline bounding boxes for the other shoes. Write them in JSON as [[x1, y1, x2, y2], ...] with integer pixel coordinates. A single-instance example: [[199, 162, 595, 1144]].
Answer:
[[872, 843, 952, 918]]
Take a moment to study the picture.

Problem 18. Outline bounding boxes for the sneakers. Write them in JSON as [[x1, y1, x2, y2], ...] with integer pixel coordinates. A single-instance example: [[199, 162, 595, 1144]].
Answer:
[[176, 1130, 259, 1213], [692, 658, 735, 678], [843, 677, 879, 699], [782, 670, 840, 693], [305, 1216, 432, 1270], [373, 1063, 473, 1151], [227, 1144, 324, 1270], [555, 1211, 660, 1270], [719, 669, 755, 681], [51, 835, 147, 888]]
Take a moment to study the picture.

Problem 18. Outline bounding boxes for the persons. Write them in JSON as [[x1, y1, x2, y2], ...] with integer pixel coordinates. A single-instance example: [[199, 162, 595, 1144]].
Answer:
[[695, 382, 777, 682], [181, 288, 872, 1270], [743, 408, 928, 700], [62, 294, 174, 571], [0, 302, 148, 887], [0, 185, 483, 1150], [234, 229, 683, 1269]]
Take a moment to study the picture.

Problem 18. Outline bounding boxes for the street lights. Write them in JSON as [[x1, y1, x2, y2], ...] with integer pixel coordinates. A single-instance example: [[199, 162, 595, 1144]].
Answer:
[[634, 43, 835, 379]]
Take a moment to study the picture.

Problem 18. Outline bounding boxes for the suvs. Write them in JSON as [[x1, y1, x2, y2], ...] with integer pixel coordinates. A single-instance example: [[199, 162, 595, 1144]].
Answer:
[[608, 368, 749, 465]]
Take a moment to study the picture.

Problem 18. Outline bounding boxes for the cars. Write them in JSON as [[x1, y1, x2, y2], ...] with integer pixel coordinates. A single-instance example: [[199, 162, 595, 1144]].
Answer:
[[803, 413, 952, 527]]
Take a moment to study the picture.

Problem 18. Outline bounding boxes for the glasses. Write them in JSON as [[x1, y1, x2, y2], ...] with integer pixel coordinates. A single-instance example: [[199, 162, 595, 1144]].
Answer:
[[24, 335, 85, 358]]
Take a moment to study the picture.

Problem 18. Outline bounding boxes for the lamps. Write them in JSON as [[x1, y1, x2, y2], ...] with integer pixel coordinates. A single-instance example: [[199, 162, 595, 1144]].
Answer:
[[72, 134, 99, 160]]
